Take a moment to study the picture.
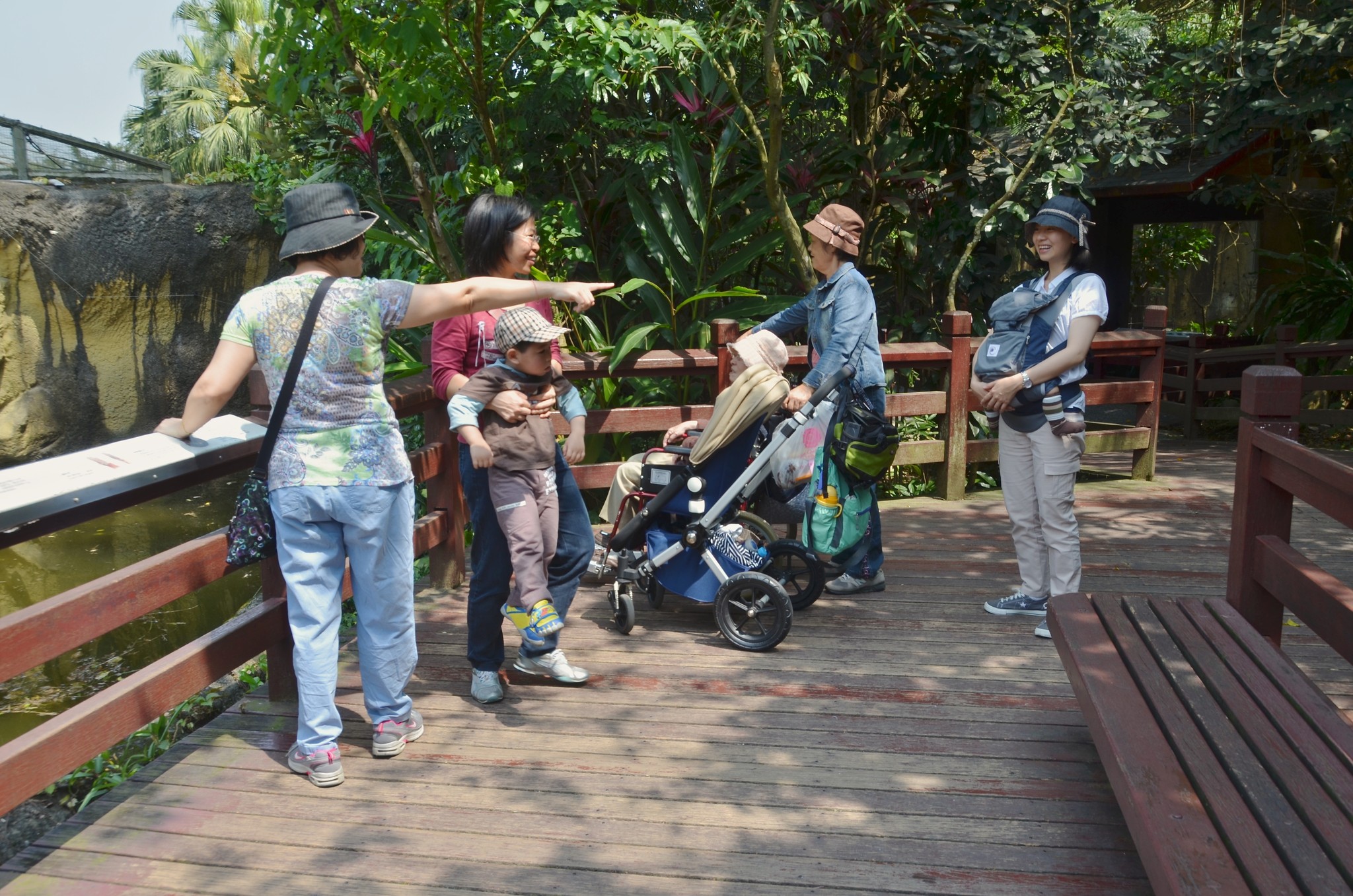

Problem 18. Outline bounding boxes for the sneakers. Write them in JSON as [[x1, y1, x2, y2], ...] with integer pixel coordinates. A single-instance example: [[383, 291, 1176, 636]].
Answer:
[[470, 668, 504, 704], [371, 708, 423, 756], [983, 587, 1050, 616], [1034, 617, 1052, 638], [513, 646, 590, 683], [285, 740, 345, 787]]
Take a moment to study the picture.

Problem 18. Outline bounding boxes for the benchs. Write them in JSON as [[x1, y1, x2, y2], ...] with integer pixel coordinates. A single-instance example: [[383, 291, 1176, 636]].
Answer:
[[1049, 363, 1353, 896]]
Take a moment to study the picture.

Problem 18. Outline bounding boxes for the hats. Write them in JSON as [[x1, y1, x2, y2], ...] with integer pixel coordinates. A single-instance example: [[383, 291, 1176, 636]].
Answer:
[[726, 329, 789, 376], [279, 182, 380, 261], [1024, 196, 1097, 250], [494, 306, 572, 353], [803, 204, 865, 256]]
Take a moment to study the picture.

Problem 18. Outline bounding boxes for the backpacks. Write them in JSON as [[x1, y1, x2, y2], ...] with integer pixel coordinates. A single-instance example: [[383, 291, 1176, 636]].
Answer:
[[802, 447, 873, 555]]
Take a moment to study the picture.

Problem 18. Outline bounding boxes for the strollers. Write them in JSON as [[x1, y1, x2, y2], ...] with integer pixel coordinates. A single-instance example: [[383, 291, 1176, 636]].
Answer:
[[595, 365, 859, 652]]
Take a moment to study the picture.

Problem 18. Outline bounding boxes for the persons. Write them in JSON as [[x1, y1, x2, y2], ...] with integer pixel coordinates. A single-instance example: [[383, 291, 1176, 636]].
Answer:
[[159, 183, 614, 791], [447, 306, 590, 652], [583, 326, 792, 554], [979, 290, 1084, 436], [968, 196, 1108, 636], [732, 201, 890, 594], [434, 192, 601, 705]]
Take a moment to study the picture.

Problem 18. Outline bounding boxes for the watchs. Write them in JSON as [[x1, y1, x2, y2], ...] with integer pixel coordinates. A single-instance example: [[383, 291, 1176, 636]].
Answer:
[[1020, 371, 1033, 390]]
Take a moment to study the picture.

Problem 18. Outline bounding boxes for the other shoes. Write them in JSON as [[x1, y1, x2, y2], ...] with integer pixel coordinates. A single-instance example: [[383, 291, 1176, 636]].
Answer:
[[529, 600, 565, 637], [500, 601, 545, 646]]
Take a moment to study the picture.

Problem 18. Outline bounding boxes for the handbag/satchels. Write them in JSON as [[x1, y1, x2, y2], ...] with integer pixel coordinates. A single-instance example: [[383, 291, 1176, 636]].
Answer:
[[821, 363, 899, 499], [225, 470, 277, 566]]
